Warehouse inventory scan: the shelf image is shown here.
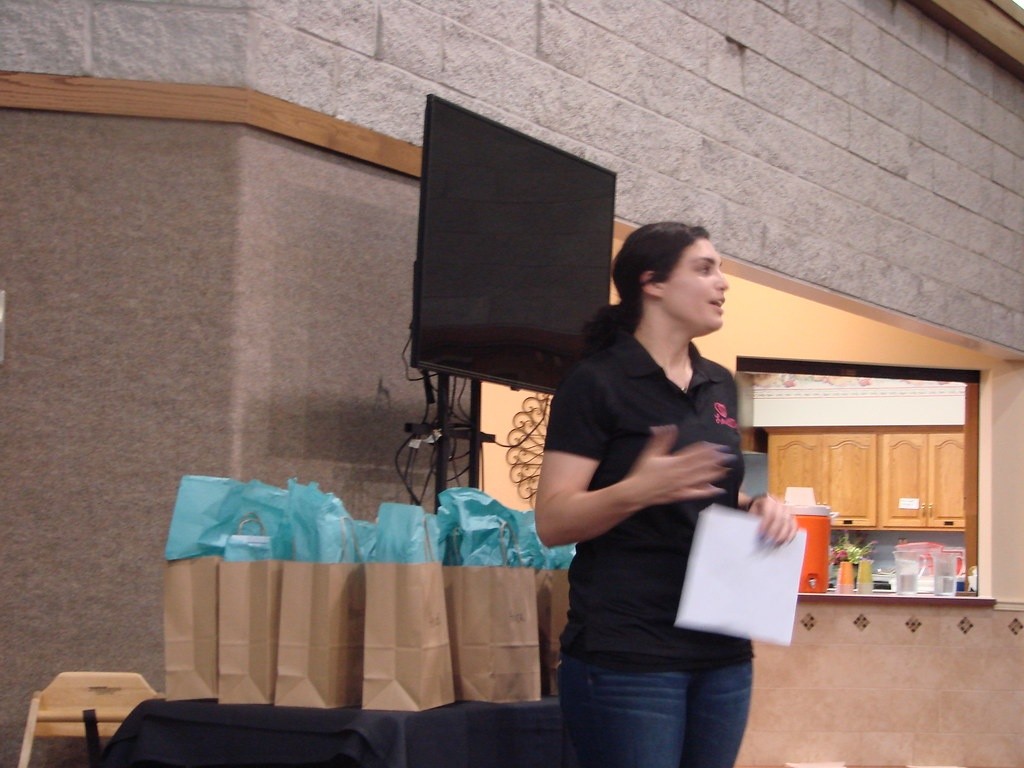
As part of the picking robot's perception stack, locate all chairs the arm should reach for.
[17,672,157,768]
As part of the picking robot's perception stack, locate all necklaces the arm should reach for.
[656,356,688,392]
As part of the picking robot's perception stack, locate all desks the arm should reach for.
[104,700,561,768]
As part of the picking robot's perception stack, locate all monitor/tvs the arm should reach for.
[409,93,617,395]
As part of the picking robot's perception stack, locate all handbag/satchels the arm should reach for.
[441,521,542,705]
[532,568,571,697]
[163,506,364,712]
[362,514,456,713]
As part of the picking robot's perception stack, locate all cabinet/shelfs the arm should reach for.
[767,424,968,533]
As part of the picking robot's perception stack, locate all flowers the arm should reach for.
[828,530,877,566]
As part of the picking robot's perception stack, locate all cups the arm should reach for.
[836,561,874,594]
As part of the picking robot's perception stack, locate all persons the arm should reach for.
[535,223,797,768]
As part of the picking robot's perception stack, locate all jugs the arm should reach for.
[931,553,964,595]
[894,542,943,594]
[892,551,928,596]
